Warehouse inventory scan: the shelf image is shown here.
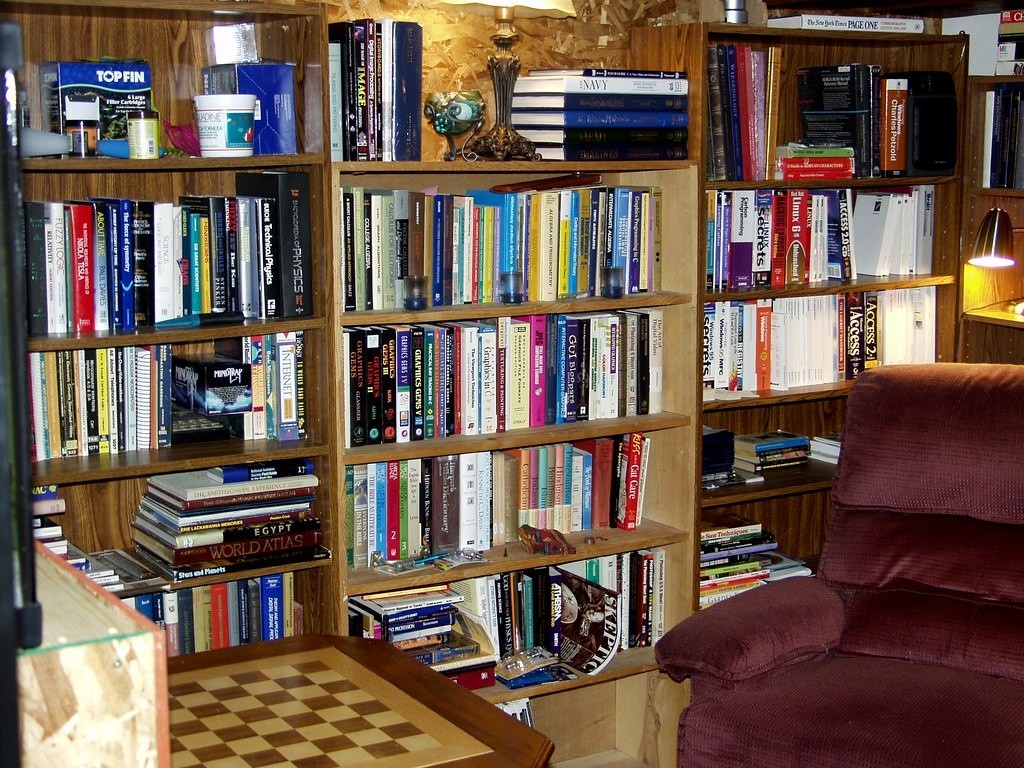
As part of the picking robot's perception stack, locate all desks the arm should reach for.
[166,634,555,768]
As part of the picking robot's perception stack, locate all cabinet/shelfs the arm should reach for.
[0,0,348,636]
[331,162,698,768]
[632,22,970,610]
[957,76,1024,364]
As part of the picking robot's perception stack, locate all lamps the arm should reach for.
[968,208,1024,316]
[428,0,577,161]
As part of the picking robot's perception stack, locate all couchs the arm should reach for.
[655,362,1024,768]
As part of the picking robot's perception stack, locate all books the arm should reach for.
[19,8,1024,691]
[528,68,687,80]
